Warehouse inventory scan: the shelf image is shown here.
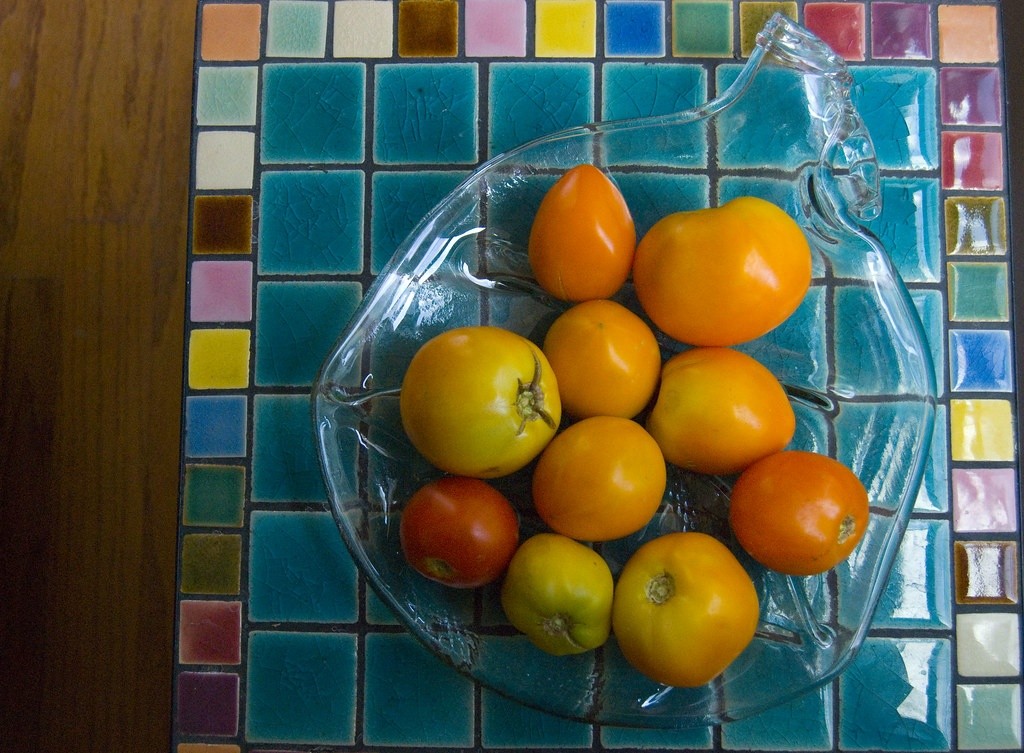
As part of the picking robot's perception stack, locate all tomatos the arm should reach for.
[401,159,869,691]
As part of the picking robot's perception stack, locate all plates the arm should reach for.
[311,14,943,727]
